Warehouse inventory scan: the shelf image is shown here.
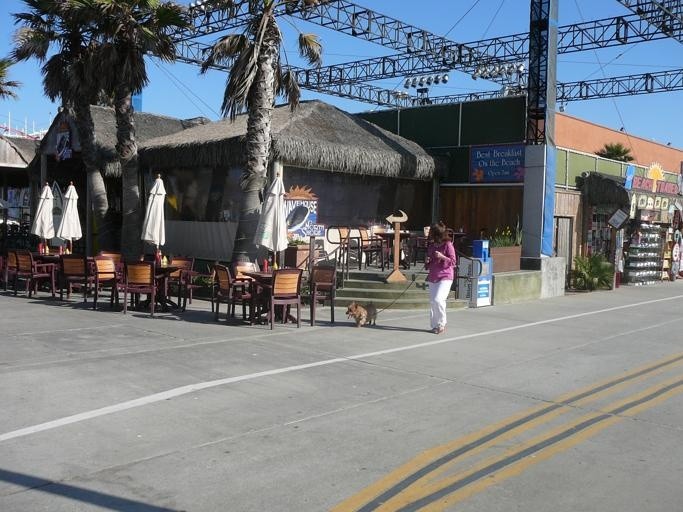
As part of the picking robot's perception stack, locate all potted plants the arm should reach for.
[489,214,523,273]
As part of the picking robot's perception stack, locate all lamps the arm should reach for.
[559,102,567,112]
[472,58,527,80]
[404,70,450,89]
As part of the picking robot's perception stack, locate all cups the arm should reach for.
[166,256,172,265]
[386,225,389,232]
[423,227,429,237]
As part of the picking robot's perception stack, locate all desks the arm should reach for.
[121,262,180,310]
[241,271,275,284]
[33,253,61,264]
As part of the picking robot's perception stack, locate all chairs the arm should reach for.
[13,249,56,298]
[233,262,261,281]
[99,250,123,268]
[124,261,163,318]
[166,257,195,307]
[301,266,338,326]
[250,267,303,330]
[4,249,30,291]
[215,264,253,325]
[93,255,134,310]
[326,225,485,271]
[59,254,96,304]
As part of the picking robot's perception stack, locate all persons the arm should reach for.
[424,220,456,334]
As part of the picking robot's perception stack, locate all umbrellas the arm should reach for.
[139,174,165,261]
[252,172,289,267]
[56,182,82,255]
[30,182,54,253]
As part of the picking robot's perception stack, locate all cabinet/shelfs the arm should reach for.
[622,221,666,285]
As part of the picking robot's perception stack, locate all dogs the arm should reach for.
[345,300,378,327]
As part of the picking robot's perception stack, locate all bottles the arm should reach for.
[153,250,160,265]
[38,242,69,255]
[162,255,167,265]
[272,262,276,270]
[262,258,267,272]
